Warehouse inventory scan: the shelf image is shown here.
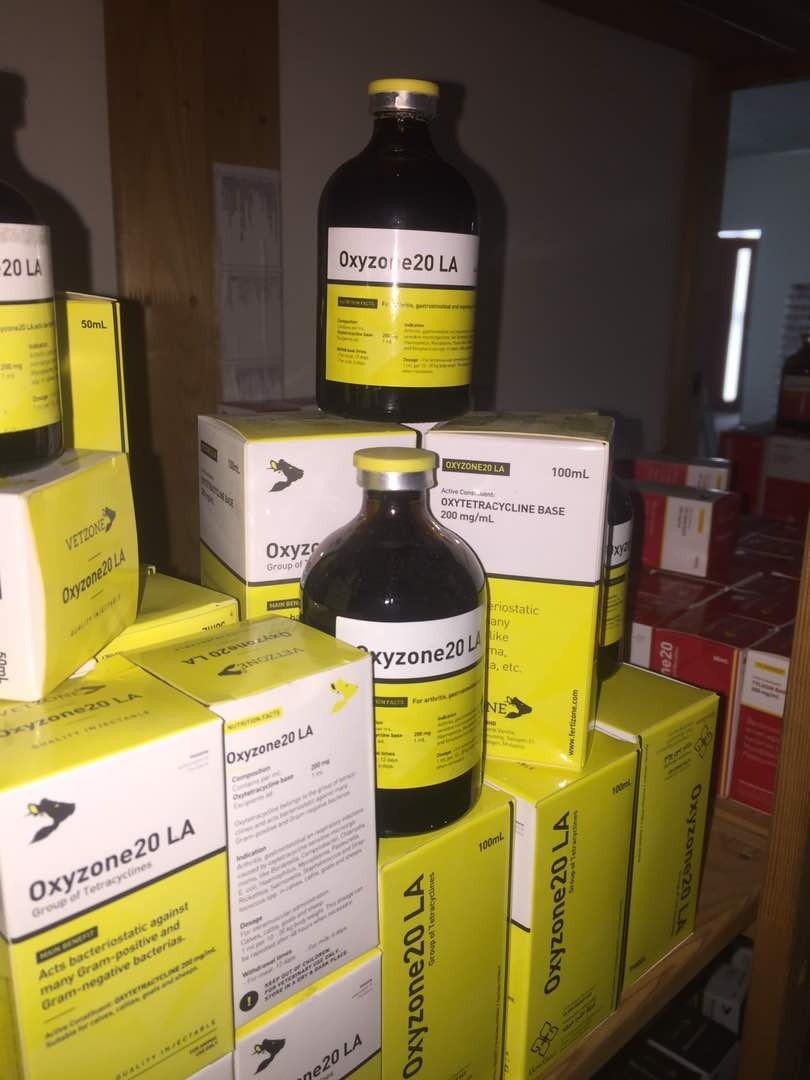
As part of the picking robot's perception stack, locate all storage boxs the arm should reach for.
[1,290,809,1079]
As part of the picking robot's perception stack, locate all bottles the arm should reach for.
[0,164,65,479]
[596,490,634,679]
[316,73,496,414]
[297,446,489,834]
[777,334,810,432]
